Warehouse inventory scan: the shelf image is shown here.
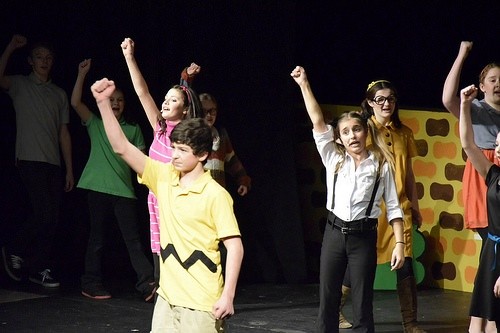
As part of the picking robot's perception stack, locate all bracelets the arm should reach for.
[396,242,404,244]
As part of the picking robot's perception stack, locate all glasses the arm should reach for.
[204,107,217,116]
[371,95,397,105]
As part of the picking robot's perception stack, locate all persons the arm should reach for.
[338,80,426,333]
[180,63,249,196]
[291,65,404,333]
[459,85,500,333]
[71,58,156,302]
[121,38,205,305]
[90,78,244,333]
[0,35,74,287]
[442,42,500,262]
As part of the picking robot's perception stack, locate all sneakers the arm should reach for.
[28,269,60,287]
[142,282,157,302]
[82,285,111,299]
[2,247,24,281]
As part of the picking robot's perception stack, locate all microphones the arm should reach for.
[161,110,163,112]
[347,144,350,146]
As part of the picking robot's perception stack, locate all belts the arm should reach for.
[328,218,377,235]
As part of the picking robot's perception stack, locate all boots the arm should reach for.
[338,285,353,329]
[397,276,427,333]
